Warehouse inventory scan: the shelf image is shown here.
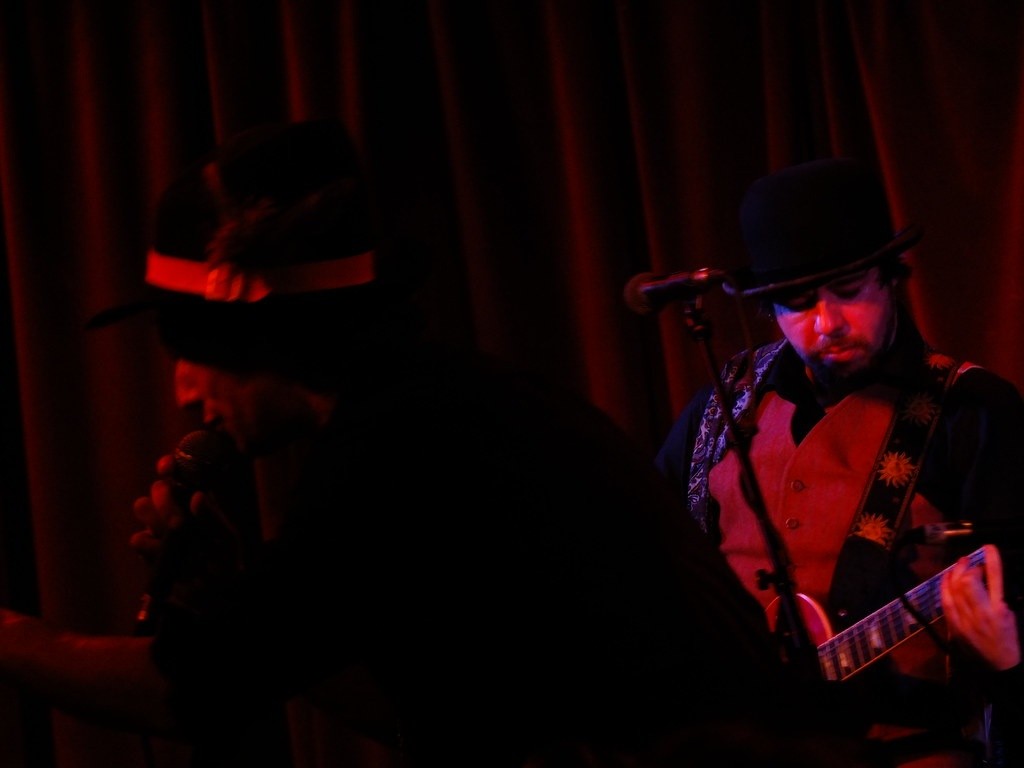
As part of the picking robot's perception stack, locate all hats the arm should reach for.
[146,121,424,390]
[720,157,924,301]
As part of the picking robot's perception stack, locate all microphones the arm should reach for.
[624,270,728,314]
[137,430,228,634]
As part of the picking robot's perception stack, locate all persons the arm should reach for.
[657,161,1024,766]
[1,119,775,766]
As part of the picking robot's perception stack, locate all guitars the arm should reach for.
[763,543,1004,684]
